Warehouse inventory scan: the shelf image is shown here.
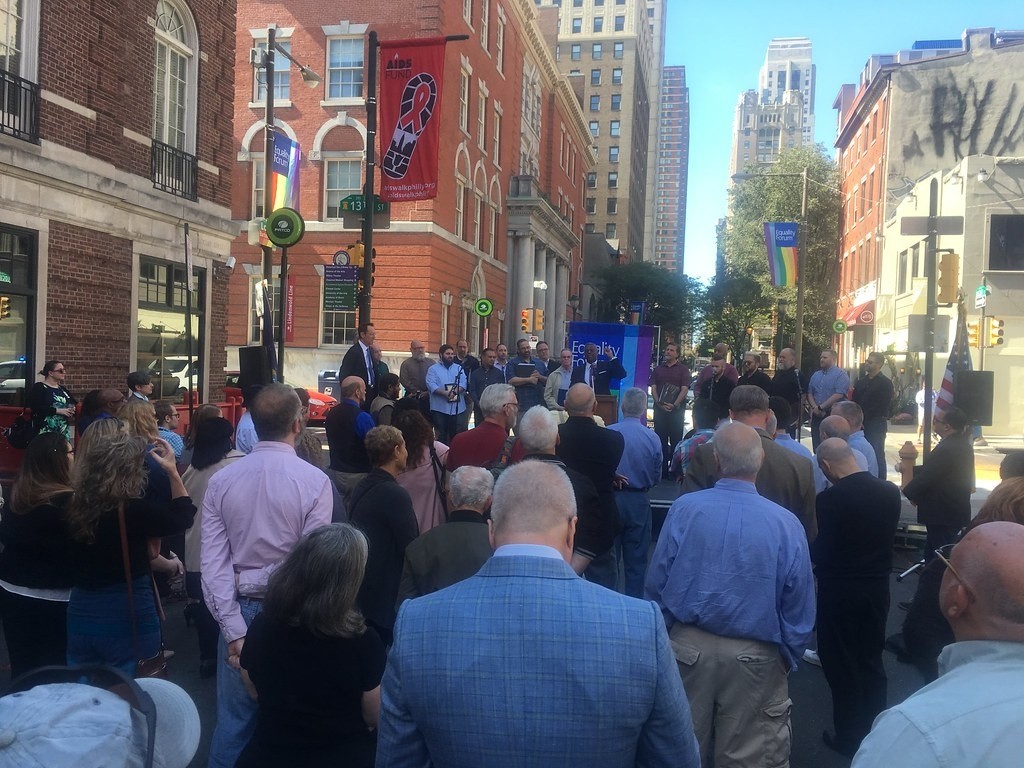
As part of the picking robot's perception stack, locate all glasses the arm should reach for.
[503,403,521,411]
[743,360,757,365]
[111,396,125,402]
[933,415,953,428]
[865,359,880,365]
[63,448,76,455]
[0,664,156,768]
[935,544,975,603]
[52,368,66,373]
[170,414,180,418]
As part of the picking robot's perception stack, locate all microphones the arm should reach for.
[460,358,468,365]
[794,369,799,374]
[591,362,596,376]
[712,367,717,375]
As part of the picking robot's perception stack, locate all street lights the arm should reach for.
[730,170,810,371]
[746,326,753,351]
[261,43,324,345]
[777,298,789,356]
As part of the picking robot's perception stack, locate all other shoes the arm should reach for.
[898,602,912,612]
[973,439,988,446]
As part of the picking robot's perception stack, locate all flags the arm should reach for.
[933,303,973,430]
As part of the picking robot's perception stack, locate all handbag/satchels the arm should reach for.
[437,462,448,518]
[490,436,517,486]
[6,383,52,448]
[138,652,168,681]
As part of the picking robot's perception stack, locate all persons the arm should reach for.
[0,322,1024,768]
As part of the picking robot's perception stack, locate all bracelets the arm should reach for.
[673,403,678,408]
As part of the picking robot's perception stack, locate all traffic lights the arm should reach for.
[991,318,1005,346]
[521,310,530,333]
[354,243,376,287]
[968,319,980,347]
[938,253,960,302]
[535,309,546,330]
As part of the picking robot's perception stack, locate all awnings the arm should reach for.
[841,299,874,331]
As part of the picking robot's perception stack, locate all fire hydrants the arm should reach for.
[894,440,919,491]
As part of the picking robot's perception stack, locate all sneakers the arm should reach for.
[802,648,823,667]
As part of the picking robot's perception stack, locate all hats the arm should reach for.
[0,677,201,768]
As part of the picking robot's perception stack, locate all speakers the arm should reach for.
[954,370,993,426]
[239,346,272,389]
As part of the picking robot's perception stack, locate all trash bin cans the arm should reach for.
[317,369,341,403]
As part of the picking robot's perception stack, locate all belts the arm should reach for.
[614,486,649,493]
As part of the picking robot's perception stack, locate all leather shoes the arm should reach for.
[823,729,854,757]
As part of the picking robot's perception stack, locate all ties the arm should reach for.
[589,365,594,388]
[367,348,375,385]
[502,365,505,381]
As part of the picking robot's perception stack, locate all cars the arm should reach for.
[686,380,697,401]
[184,371,335,427]
[0,361,26,394]
[147,355,198,403]
